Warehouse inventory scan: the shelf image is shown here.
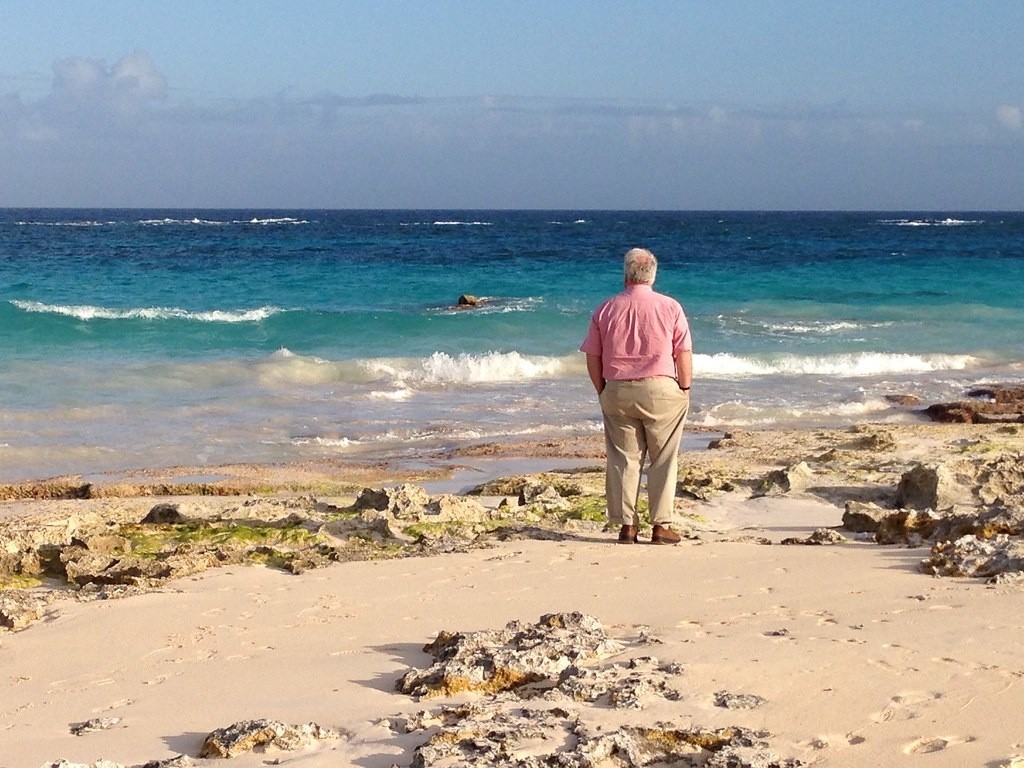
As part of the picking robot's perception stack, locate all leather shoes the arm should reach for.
[652,525,681,544]
[619,525,639,543]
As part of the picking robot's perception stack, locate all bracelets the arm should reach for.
[680,386,691,390]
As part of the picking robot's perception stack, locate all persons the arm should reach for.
[580,246,693,544]
